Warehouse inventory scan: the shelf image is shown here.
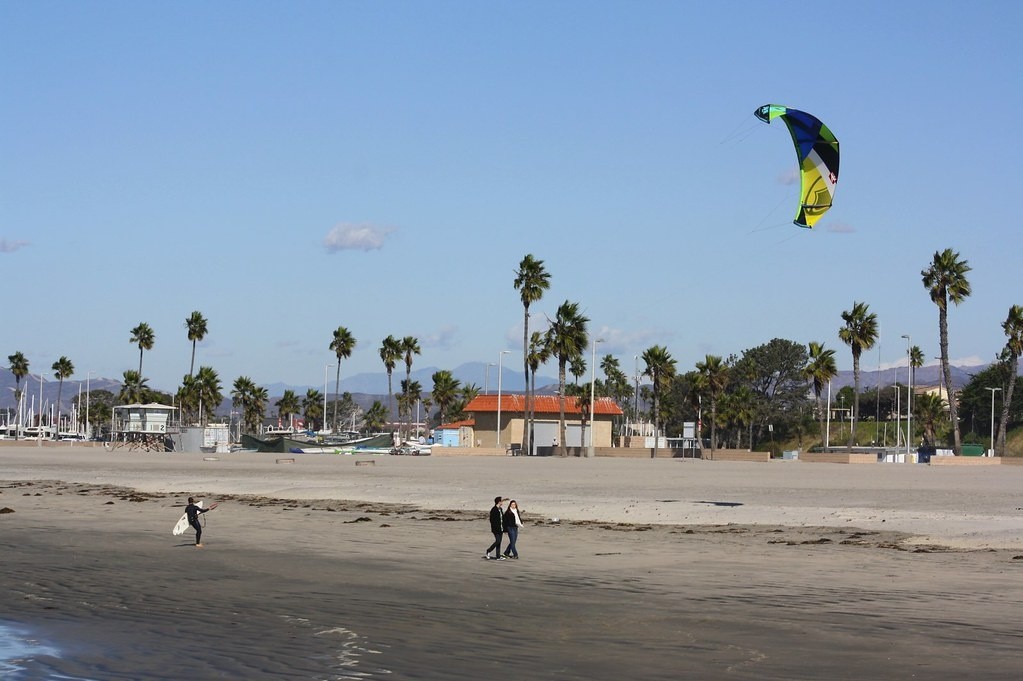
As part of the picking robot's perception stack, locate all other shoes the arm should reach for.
[502,554,510,558]
[511,556,518,559]
[485,552,491,560]
[196,544,202,547]
[496,556,506,561]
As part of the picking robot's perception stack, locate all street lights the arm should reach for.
[901,335,911,454]
[590,339,608,447]
[485,363,497,395]
[891,386,900,448]
[86,372,95,436]
[497,350,511,444]
[38,373,48,445]
[324,365,335,430]
[985,387,1002,457]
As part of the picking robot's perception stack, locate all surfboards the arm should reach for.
[172,501,205,537]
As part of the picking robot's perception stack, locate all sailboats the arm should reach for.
[0,380,87,442]
[343,412,360,439]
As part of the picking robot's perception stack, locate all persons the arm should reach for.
[418,435,434,445]
[484,497,507,560]
[185,497,210,547]
[502,500,524,559]
[552,437,559,447]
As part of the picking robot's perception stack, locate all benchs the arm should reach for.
[506,444,522,456]
